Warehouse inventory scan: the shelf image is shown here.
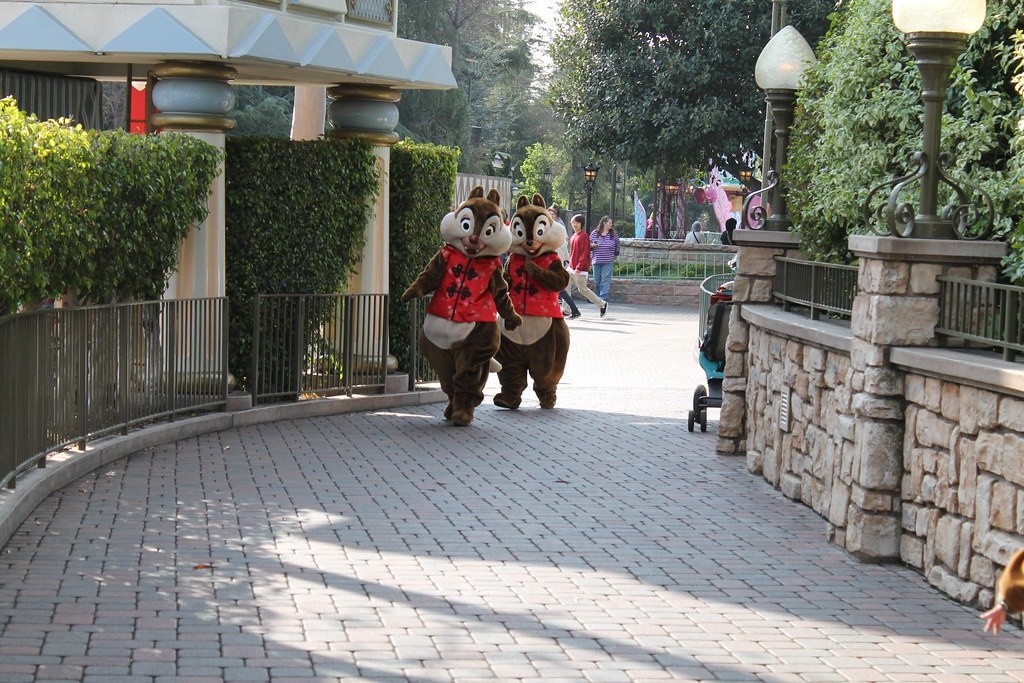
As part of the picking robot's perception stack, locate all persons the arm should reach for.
[587,216,620,304]
[499,207,581,319]
[648,215,658,229]
[562,215,608,318]
[685,221,706,243]
[720,218,737,245]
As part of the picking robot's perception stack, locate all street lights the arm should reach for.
[664,179,680,240]
[737,163,754,229]
[583,162,599,236]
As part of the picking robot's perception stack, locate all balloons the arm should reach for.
[692,187,717,204]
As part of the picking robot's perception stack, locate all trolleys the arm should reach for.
[688,273,738,432]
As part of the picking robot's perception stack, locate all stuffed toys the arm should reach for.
[402,186,569,425]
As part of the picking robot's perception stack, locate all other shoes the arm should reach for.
[599,302,608,318]
[565,313,581,320]
[562,311,570,317]
